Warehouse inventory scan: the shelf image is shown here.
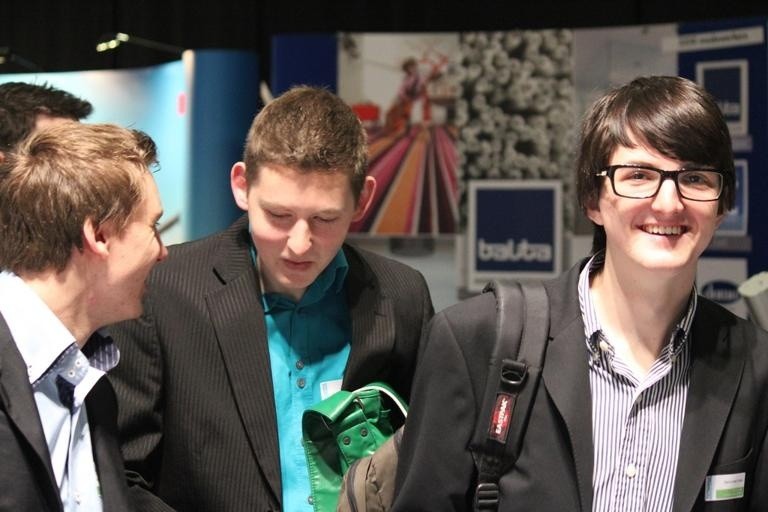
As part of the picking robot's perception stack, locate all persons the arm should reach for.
[0,123,169,512]
[100,84,434,512]
[395,73,768,510]
[0,81,93,164]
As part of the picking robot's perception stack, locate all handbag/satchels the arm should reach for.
[300,380,410,512]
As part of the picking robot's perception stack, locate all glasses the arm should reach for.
[596,163,724,203]
